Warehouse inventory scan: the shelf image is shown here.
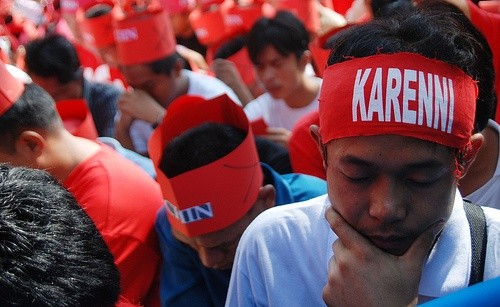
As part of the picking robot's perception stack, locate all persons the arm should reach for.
[146,93,328,307]
[1,163,122,307]
[224,13,500,307]
[0,0,500,210]
[1,63,163,307]
